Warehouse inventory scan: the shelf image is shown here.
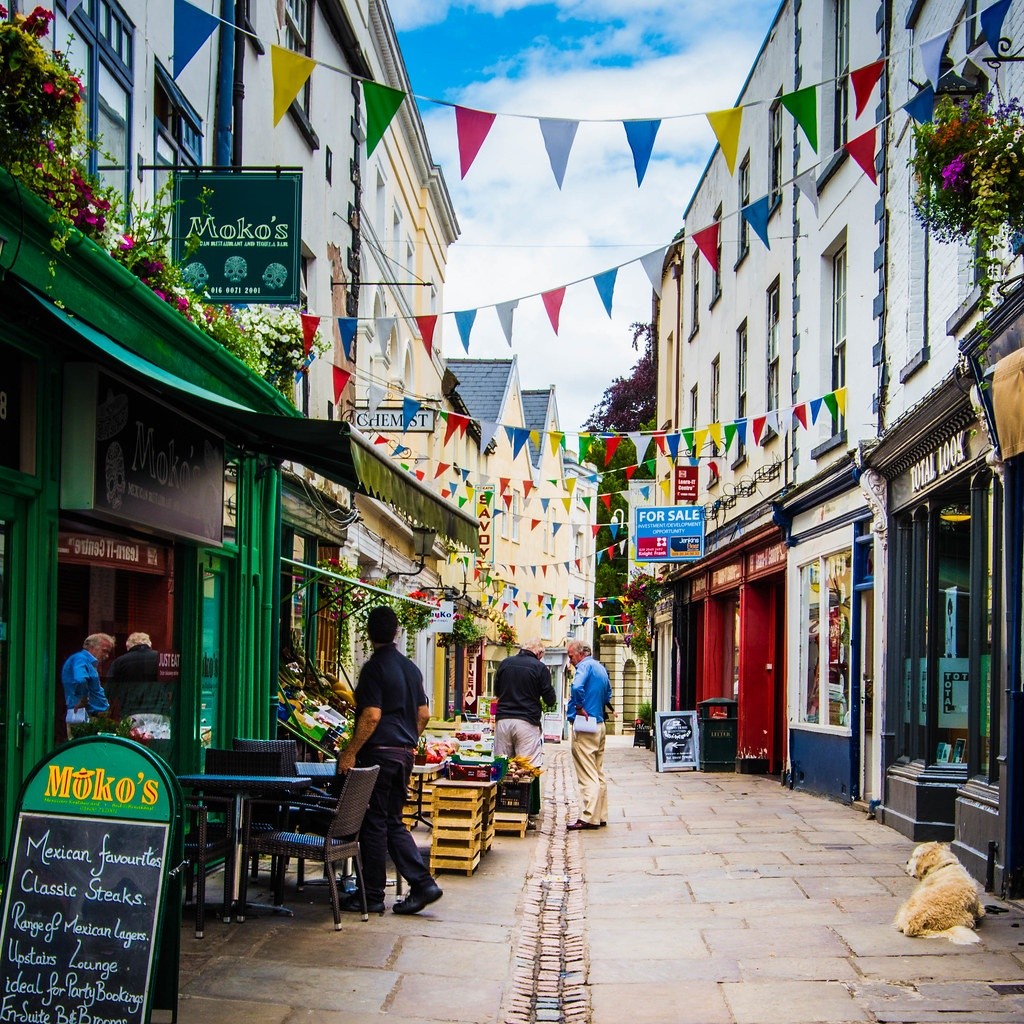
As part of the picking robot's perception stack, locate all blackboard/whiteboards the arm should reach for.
[0,811,179,1023]
[634,723,650,746]
[655,711,700,767]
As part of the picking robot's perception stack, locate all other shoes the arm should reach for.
[528,813,540,821]
[331,893,386,913]
[392,886,444,915]
[566,819,599,830]
[600,821,607,827]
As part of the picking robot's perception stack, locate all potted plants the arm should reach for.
[415,736,428,766]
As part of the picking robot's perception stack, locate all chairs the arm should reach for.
[181,736,380,931]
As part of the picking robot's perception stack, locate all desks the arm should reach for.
[403,760,445,831]
[180,774,311,921]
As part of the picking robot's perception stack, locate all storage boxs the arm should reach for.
[495,781,534,813]
[278,688,355,756]
[446,762,492,782]
[451,753,510,778]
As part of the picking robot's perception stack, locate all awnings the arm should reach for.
[16,279,482,556]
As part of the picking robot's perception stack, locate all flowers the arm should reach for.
[904,90,1024,455]
[0,4,333,405]
[622,566,663,681]
[295,557,485,679]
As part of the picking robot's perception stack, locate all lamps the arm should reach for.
[940,505,971,522]
[387,523,438,580]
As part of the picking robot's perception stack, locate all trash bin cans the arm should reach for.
[697,697,738,773]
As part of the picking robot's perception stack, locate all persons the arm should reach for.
[492,638,556,829]
[59,632,115,740]
[563,642,612,830]
[604,703,619,721]
[337,605,443,916]
[106,631,178,713]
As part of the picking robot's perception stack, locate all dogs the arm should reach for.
[894,841,986,946]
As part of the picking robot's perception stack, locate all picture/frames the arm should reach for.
[951,739,967,763]
